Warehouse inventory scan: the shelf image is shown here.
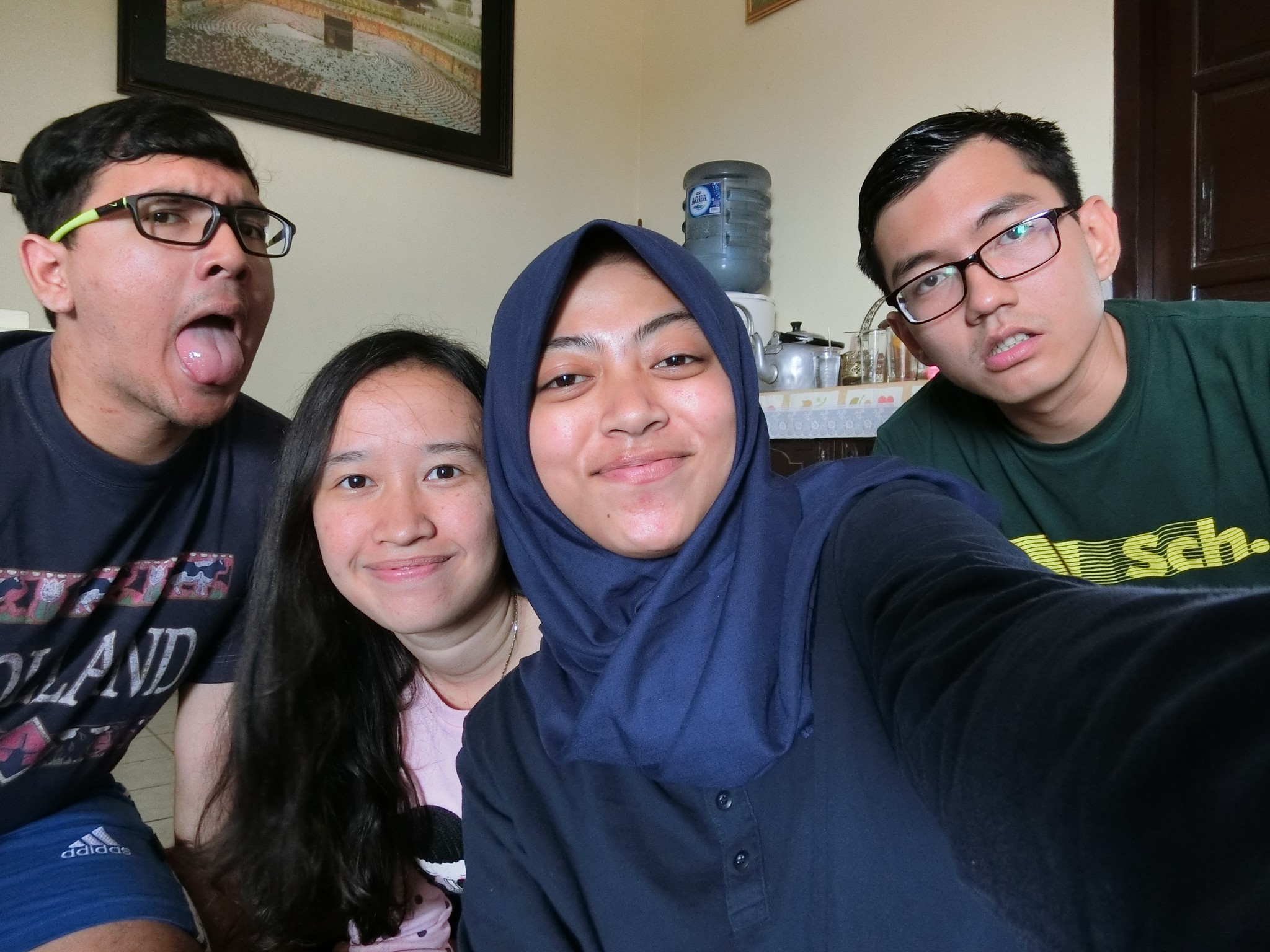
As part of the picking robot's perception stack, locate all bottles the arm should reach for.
[679,161,770,291]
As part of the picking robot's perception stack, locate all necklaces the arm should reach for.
[493,585,525,683]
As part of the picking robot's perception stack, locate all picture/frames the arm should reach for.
[113,0,515,180]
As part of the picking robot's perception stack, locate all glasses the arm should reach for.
[48,192,297,262]
[884,204,1079,326]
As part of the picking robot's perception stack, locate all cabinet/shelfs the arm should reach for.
[755,378,939,481]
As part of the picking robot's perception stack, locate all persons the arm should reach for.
[855,105,1268,592]
[454,221,1268,952]
[2,96,306,950]
[198,322,554,950]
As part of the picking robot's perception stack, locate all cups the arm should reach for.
[861,330,892,384]
[811,346,844,389]
[888,328,920,381]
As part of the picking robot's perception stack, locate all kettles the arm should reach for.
[750,322,845,391]
